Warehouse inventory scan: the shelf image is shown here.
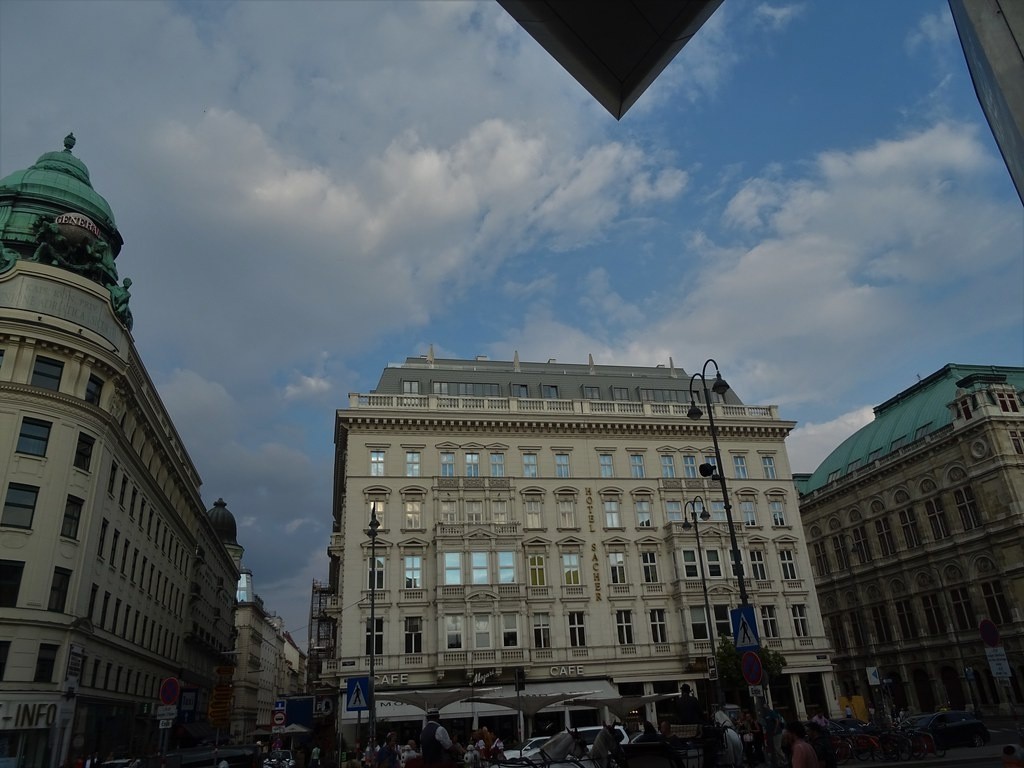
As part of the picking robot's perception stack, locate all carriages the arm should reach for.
[404,720,626,768]
[620,706,744,768]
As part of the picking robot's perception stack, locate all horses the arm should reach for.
[711,704,743,768]
[540,718,625,768]
[487,726,589,768]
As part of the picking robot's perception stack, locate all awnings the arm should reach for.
[185,721,218,737]
[340,679,622,724]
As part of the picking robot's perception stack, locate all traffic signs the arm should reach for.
[208,666,234,725]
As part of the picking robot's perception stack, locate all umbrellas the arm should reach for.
[247,728,272,735]
[285,723,312,750]
[371,689,680,736]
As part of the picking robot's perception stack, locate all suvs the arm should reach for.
[262,750,296,768]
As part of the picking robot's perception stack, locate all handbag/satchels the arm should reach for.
[318,759,320,765]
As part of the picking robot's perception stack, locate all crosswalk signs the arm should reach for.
[346,677,370,712]
[729,606,761,651]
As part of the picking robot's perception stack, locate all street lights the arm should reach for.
[682,495,725,710]
[367,501,380,751]
[686,359,749,604]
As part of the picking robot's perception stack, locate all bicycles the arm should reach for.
[831,722,945,764]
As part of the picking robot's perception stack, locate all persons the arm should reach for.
[807,722,837,768]
[311,744,321,768]
[845,705,852,718]
[674,684,704,722]
[660,721,681,749]
[452,734,461,751]
[472,729,507,768]
[783,721,819,768]
[107,752,115,761]
[812,711,829,727]
[63,752,102,768]
[376,732,402,768]
[731,706,787,768]
[891,705,911,723]
[422,708,456,764]
[633,721,662,743]
[401,740,422,762]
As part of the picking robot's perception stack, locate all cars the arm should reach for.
[900,711,991,750]
[801,717,881,735]
[503,726,694,760]
[99,745,262,768]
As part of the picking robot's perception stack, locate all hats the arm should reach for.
[424,707,441,716]
